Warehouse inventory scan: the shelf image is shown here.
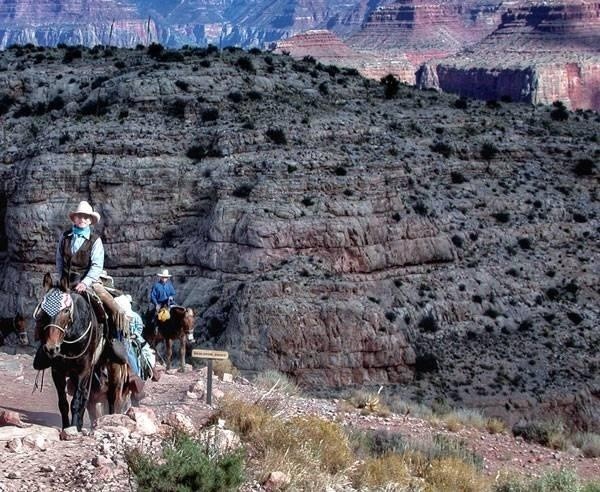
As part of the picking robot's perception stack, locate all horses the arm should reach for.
[142,304,196,374]
[40,269,124,432]
[0,312,29,346]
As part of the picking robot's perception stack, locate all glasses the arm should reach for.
[77,215,88,219]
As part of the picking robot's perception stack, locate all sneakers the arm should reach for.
[112,338,129,364]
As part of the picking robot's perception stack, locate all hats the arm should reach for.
[114,294,133,311]
[69,201,101,223]
[157,269,172,278]
[100,270,113,286]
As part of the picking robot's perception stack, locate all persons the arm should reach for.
[149,269,178,336]
[54,200,160,399]
